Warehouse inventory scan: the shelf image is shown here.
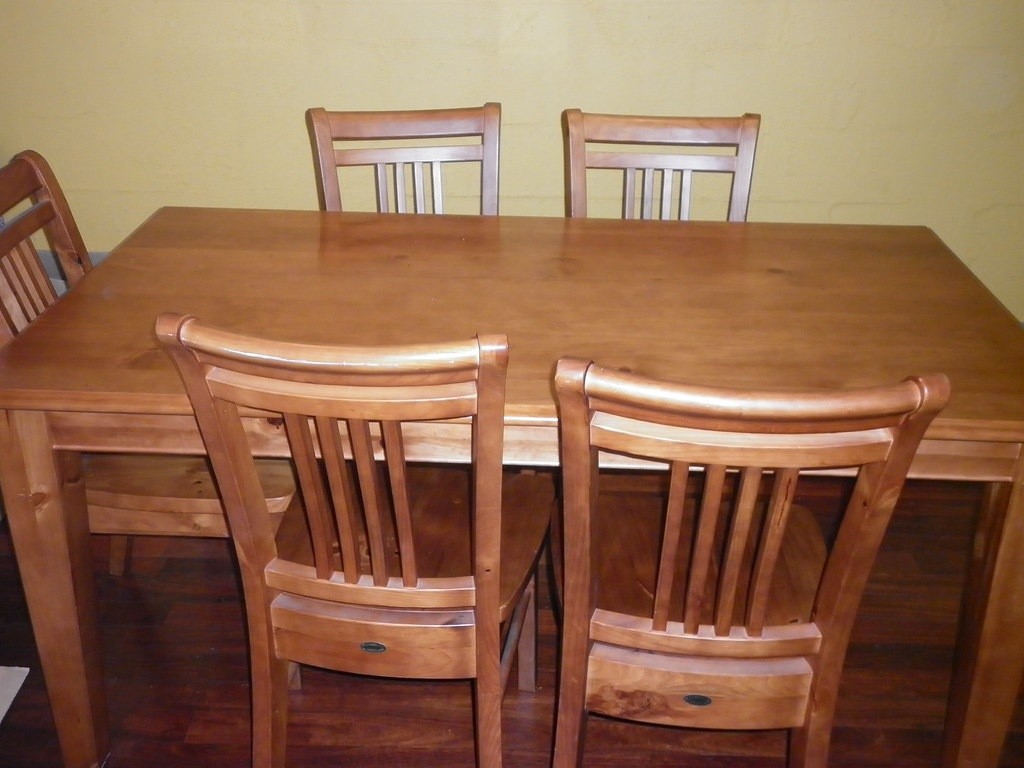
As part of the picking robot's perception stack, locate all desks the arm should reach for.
[0,204,1024,768]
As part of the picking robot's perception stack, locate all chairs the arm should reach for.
[146,310,558,768]
[555,109,762,223]
[544,352,957,768]
[303,100,509,214]
[0,147,303,698]
